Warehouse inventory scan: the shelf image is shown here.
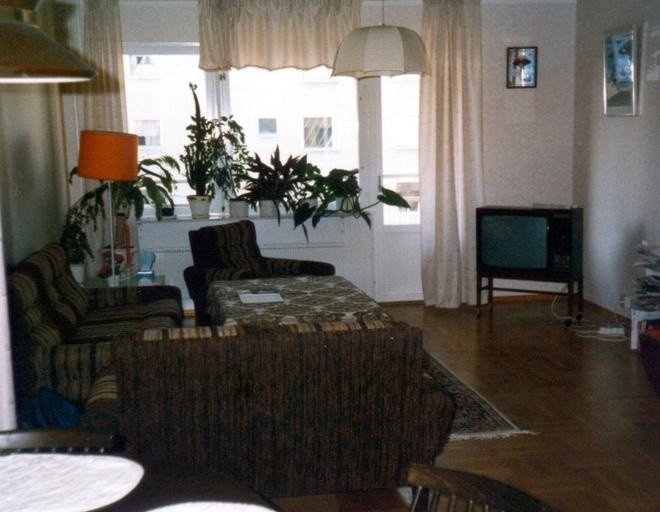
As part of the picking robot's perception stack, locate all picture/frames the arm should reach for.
[600,24,640,118]
[505,46,538,90]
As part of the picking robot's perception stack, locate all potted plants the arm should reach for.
[61,154,181,283]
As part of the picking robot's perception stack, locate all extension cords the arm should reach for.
[599,325,624,334]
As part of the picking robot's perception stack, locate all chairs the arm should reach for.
[183,219,336,326]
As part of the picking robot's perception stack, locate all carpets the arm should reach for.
[423,348,543,442]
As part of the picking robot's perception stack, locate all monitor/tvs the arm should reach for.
[476,206,583,280]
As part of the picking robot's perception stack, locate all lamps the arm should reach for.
[77,129,140,281]
[329,0,434,82]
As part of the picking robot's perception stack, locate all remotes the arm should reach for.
[533,203,572,210]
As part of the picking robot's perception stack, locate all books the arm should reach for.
[632,239,659,314]
